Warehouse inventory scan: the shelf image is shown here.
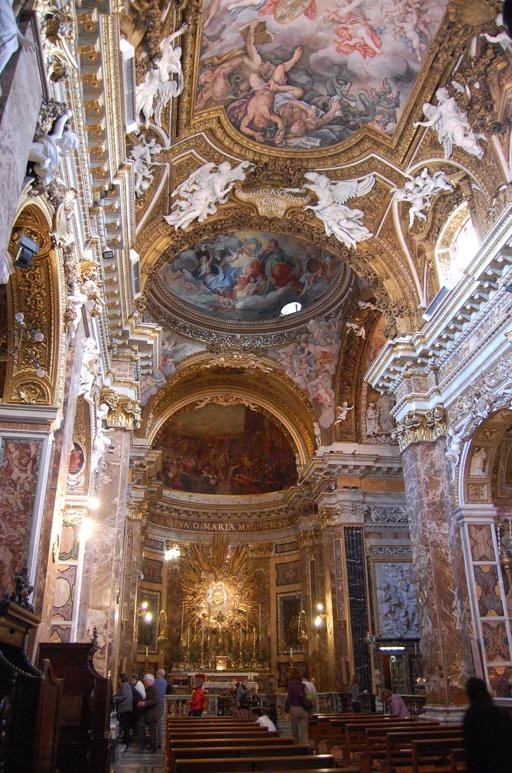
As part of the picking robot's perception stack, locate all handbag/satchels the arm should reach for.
[203,698,210,710]
[303,687,317,708]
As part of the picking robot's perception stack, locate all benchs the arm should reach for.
[163,710,469,771]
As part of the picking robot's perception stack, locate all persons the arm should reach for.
[1,493,41,597]
[412,86,487,161]
[374,560,421,639]
[459,674,511,770]
[111,667,410,753]
